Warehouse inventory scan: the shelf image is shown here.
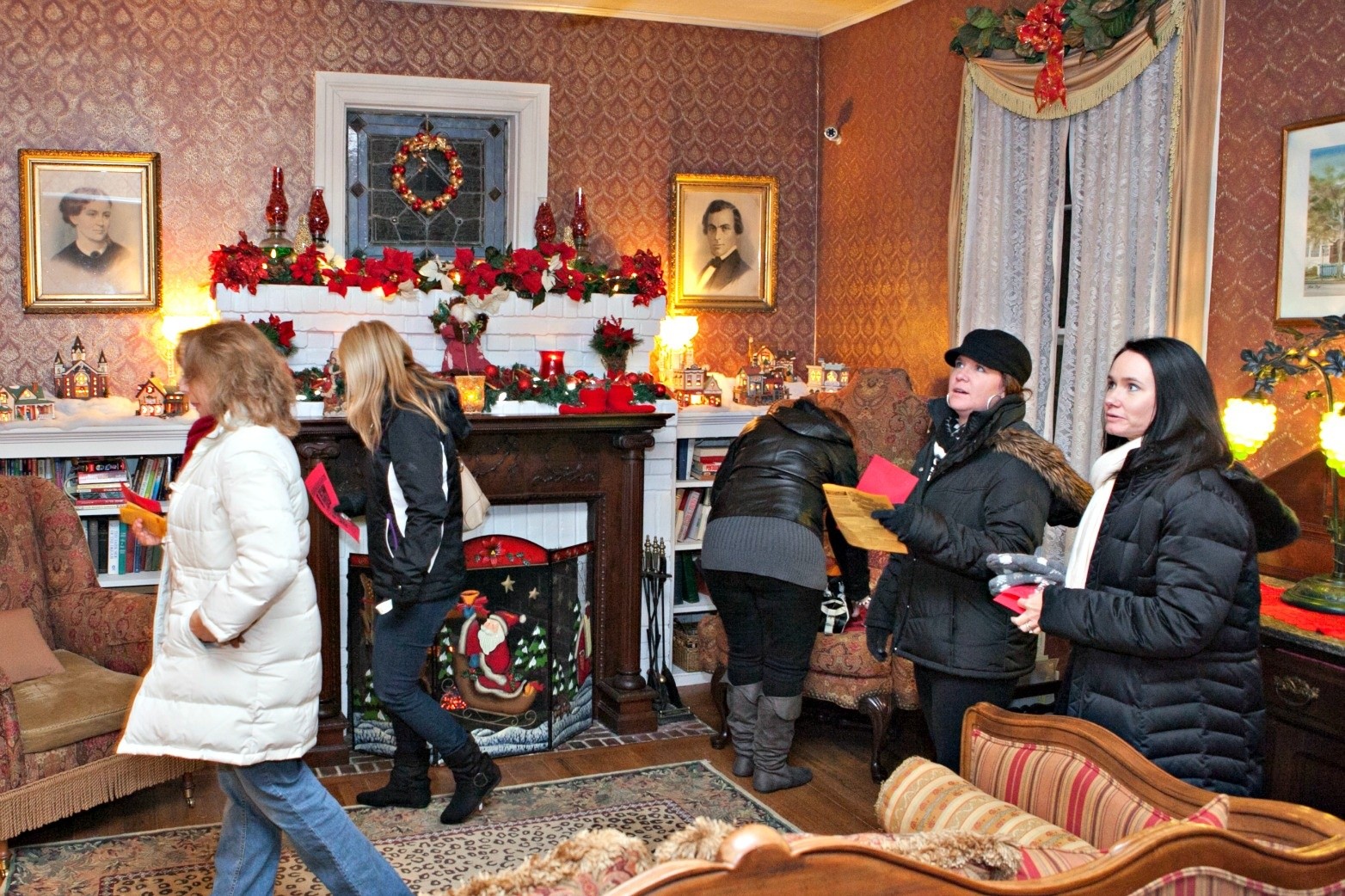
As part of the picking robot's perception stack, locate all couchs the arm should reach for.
[605,700,1345,896]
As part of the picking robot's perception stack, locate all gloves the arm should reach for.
[866,628,890,662]
[986,546,1068,579]
[872,503,914,538]
[989,572,1057,595]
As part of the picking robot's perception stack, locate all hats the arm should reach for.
[945,328,1033,388]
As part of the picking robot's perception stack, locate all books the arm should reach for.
[82,520,164,575]
[674,552,708,605]
[0,456,180,510]
[676,488,712,544]
[676,438,735,481]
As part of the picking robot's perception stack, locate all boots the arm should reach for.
[753,688,813,792]
[719,669,763,777]
[441,730,501,824]
[356,748,432,808]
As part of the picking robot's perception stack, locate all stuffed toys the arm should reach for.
[440,298,488,373]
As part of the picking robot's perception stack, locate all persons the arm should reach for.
[51,187,130,274]
[332,320,503,825]
[698,199,751,290]
[701,397,870,793]
[117,318,412,896]
[1011,337,1301,797]
[864,329,1093,770]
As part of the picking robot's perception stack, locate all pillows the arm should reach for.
[432,757,1234,896]
[0,607,66,683]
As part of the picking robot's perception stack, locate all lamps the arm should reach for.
[1219,315,1345,616]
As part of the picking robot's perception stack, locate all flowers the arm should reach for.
[204,228,670,310]
[241,312,299,360]
[590,316,644,359]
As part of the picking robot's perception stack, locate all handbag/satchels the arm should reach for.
[457,455,490,533]
[817,576,853,636]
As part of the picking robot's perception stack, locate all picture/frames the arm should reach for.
[17,147,163,315]
[1274,113,1345,327]
[665,173,781,314]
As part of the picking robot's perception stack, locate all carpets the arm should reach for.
[0,759,805,896]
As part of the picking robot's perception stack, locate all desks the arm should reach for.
[1009,656,1063,717]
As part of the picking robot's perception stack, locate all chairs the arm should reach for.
[0,474,210,878]
[696,367,921,785]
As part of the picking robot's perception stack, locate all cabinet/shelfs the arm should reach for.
[673,414,766,686]
[0,440,190,597]
[1257,643,1345,821]
